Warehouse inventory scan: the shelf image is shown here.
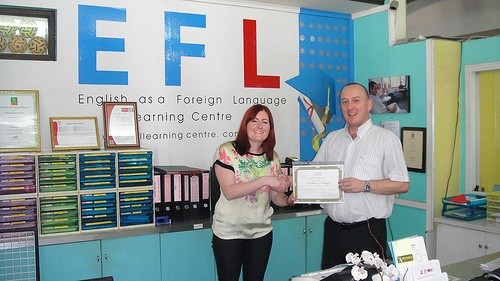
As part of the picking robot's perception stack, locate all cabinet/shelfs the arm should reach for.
[38,209,329,281]
[433,216,500,266]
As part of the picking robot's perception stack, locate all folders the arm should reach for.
[154,165,201,175]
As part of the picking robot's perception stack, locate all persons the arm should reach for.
[312,83,410,272]
[369,81,406,113]
[210,104,296,281]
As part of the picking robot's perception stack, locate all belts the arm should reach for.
[330,218,377,233]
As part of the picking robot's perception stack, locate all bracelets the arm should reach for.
[286,196,294,206]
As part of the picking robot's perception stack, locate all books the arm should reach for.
[388,236,448,281]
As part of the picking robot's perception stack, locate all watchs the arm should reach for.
[364,180,371,193]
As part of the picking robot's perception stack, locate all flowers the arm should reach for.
[346,250,400,281]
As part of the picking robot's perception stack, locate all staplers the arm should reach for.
[156,216,171,225]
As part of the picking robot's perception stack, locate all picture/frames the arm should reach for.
[0,4,58,62]
